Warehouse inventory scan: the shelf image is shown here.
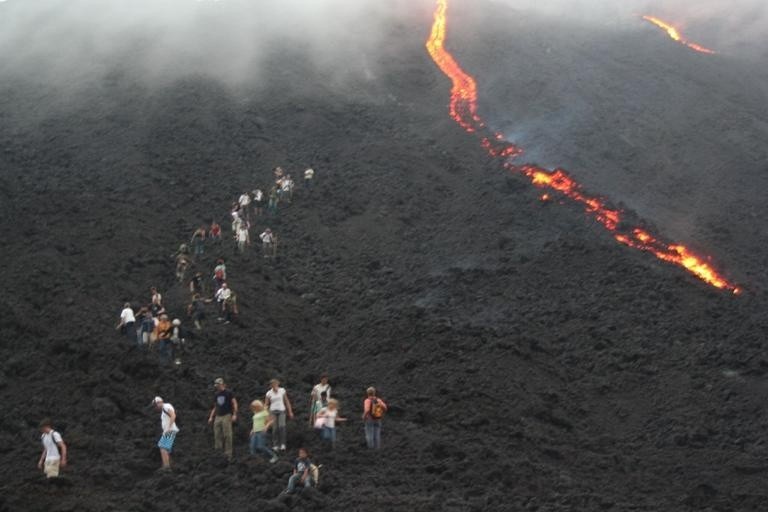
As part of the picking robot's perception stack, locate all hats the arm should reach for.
[214,378,224,387]
[39,417,50,427]
[152,396,162,404]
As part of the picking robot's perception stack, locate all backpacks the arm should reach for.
[216,268,222,280]
[369,396,384,419]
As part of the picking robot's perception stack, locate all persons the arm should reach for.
[36,421,68,479]
[209,375,391,495]
[117,165,316,365]
[152,395,180,475]
